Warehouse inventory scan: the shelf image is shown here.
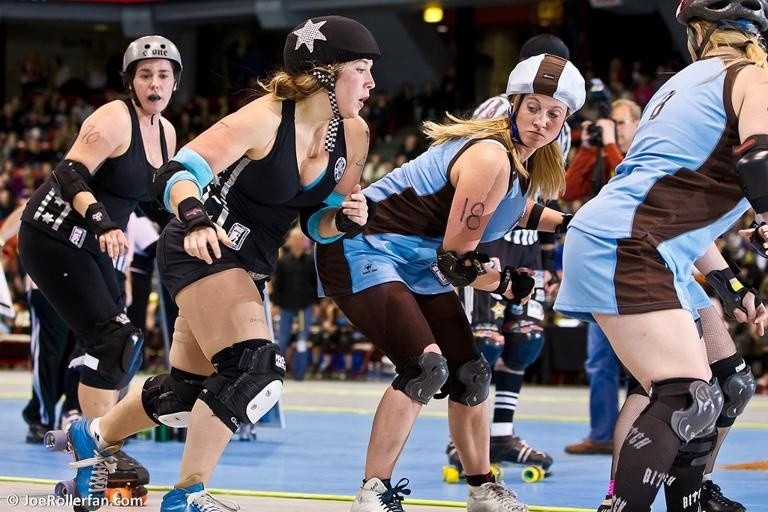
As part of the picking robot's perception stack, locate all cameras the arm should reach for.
[586,78,613,147]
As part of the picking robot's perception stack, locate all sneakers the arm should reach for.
[701,481,746,512]
[565,439,613,454]
[467,482,529,512]
[161,474,240,512]
[350,477,410,512]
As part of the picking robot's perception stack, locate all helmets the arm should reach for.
[122,36,183,89]
[284,16,381,76]
[676,0,768,31]
[506,35,586,113]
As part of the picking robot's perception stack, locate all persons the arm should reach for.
[0,0,768,512]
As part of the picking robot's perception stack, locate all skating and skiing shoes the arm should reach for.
[27,409,149,512]
[442,435,553,483]
